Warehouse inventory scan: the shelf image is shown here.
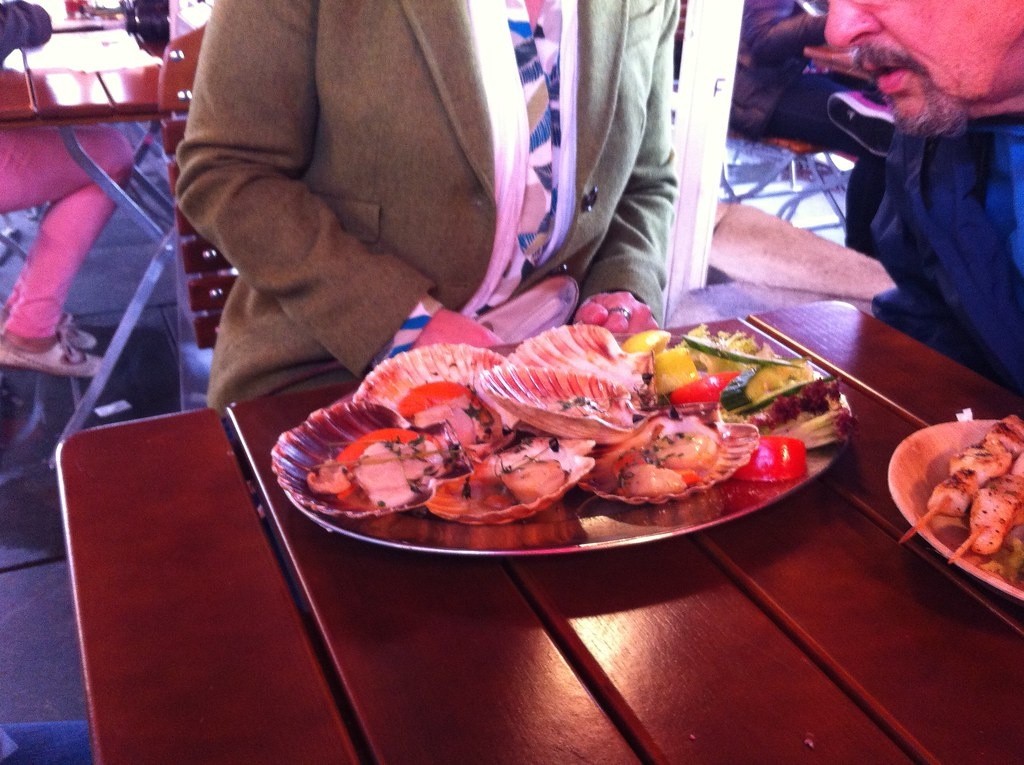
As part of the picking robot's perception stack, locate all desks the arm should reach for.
[53,298,1024,764]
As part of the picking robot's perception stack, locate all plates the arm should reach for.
[270,363,851,556]
[888,418,1024,601]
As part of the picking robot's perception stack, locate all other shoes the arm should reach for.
[0,331,101,377]
[56,311,97,350]
[827,90,898,155]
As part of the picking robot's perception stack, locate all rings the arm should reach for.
[608,306,633,320]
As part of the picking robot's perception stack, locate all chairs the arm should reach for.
[156,18,239,347]
[0,29,169,471]
[715,131,848,244]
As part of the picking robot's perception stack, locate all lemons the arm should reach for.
[655,348,699,395]
[621,330,672,356]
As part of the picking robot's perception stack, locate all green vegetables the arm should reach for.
[750,377,864,447]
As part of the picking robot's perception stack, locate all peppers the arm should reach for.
[729,436,810,484]
[673,373,738,408]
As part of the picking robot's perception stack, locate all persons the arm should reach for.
[174,0,680,420]
[730,0,1024,399]
[0,0,133,378]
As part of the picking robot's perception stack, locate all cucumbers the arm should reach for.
[683,333,841,416]
